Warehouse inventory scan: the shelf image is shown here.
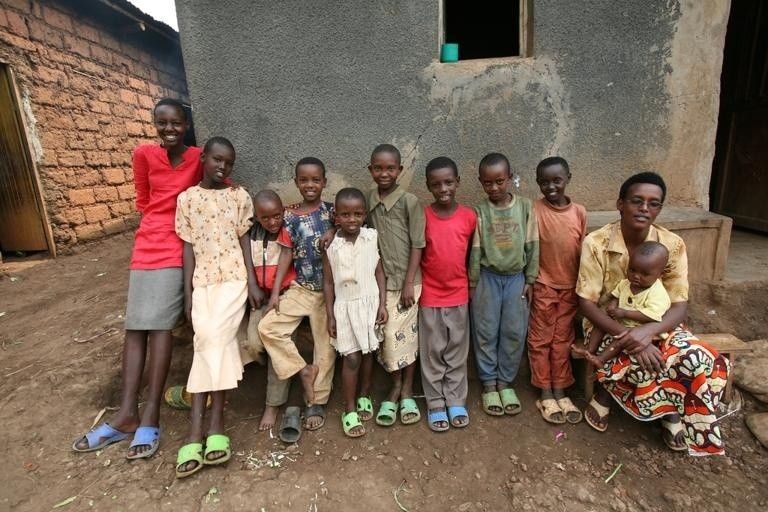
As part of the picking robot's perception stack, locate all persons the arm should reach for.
[255,155,337,432]
[468,152,541,417]
[570,170,732,456]
[530,154,587,426]
[417,156,479,432]
[317,143,428,427]
[569,240,674,370]
[173,136,267,479]
[71,98,233,461]
[320,187,391,438]
[243,187,296,432]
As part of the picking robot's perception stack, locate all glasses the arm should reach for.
[625,197,663,210]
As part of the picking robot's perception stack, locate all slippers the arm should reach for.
[535,399,566,424]
[305,404,326,430]
[499,389,521,414]
[279,407,301,443]
[165,386,212,409]
[357,395,373,421]
[342,412,365,437]
[73,422,134,452]
[557,397,582,424]
[204,435,230,464]
[176,443,203,479]
[661,417,687,450]
[400,399,420,424]
[481,392,504,416]
[376,401,399,426]
[585,395,610,432]
[428,407,449,431]
[127,427,160,459]
[448,406,468,427]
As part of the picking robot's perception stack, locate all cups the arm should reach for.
[440,42,459,63]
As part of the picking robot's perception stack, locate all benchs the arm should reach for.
[571,332,754,404]
